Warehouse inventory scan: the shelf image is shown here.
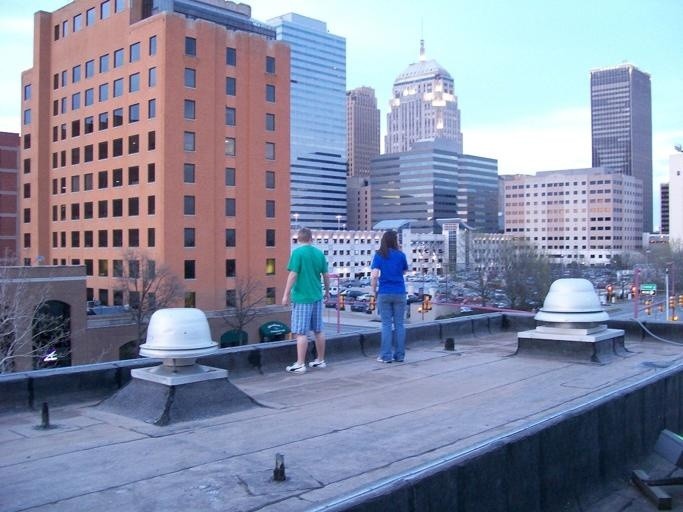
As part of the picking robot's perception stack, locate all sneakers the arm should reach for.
[375,354,404,363]
[307,358,326,368]
[284,361,306,373]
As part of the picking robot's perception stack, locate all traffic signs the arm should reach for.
[641,290,656,296]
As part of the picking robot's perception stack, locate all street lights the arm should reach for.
[293,214,299,229]
[336,215,345,230]
[665,267,669,320]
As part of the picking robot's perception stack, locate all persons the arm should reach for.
[369,230,408,364]
[280,226,331,373]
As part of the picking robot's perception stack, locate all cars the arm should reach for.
[403,270,634,312]
[322,276,378,312]
